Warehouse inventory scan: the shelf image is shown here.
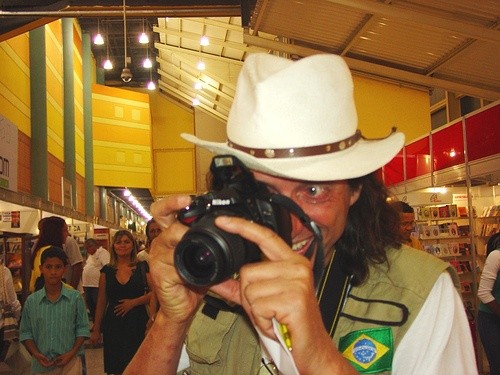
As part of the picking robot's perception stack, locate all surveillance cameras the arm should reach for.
[121,69,132,82]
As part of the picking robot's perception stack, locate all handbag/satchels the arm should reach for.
[4,308,19,342]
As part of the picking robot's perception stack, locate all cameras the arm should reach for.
[174,154,293,288]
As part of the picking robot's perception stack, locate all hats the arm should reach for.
[180,51,406,182]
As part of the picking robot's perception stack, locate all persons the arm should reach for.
[1,215,165,375]
[392,200,426,252]
[477,248,500,375]
[119,52,480,375]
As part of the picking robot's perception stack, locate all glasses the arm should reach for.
[256,357,278,374]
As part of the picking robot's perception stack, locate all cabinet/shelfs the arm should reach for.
[412,204,478,322]
[473,215,500,270]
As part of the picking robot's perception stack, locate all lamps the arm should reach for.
[143,43,152,69]
[94,17,104,45]
[104,22,113,69]
[139,18,149,43]
[147,68,155,91]
